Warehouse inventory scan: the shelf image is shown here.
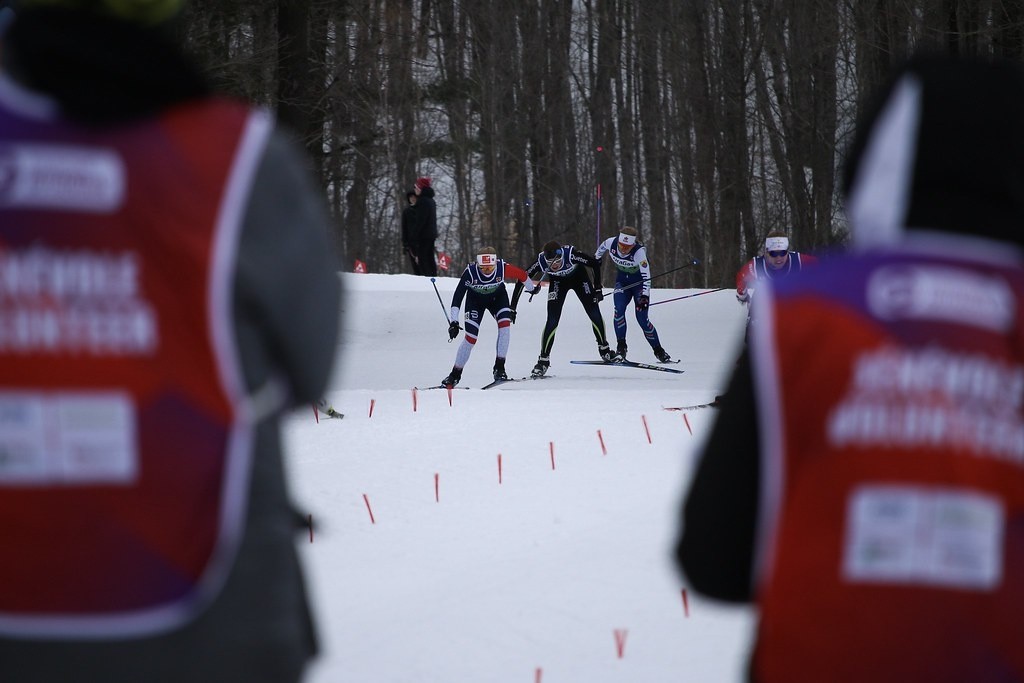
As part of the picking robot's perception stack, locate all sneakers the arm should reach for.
[441,365,463,388]
[531,353,550,378]
[654,348,671,364]
[615,343,628,358]
[492,358,507,381]
[597,342,616,363]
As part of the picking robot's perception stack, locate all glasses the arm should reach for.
[479,266,496,270]
[767,250,789,257]
[618,242,635,249]
[544,248,564,265]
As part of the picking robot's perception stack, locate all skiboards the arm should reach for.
[414,378,524,392]
[517,360,685,384]
[662,403,713,412]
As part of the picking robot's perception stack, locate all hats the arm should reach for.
[415,176,431,191]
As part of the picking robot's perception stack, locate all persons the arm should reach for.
[441,246,541,387]
[594,226,671,362]
[0,0,345,683]
[401,178,437,277]
[736,231,819,307]
[677,50,1024,683]
[510,241,615,377]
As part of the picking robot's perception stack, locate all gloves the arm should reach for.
[509,307,518,324]
[593,289,604,303]
[529,285,541,295]
[736,292,750,305]
[448,320,464,339]
[636,295,650,312]
